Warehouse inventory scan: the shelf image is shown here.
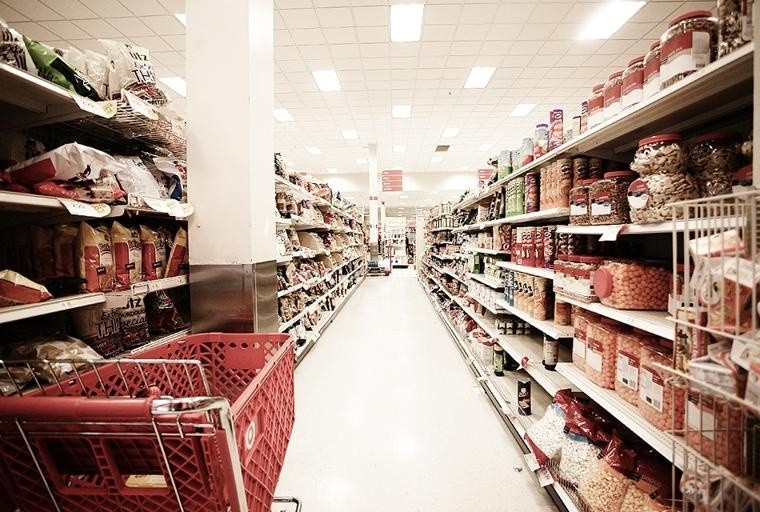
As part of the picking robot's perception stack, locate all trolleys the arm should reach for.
[366,244,392,276]
[0,329,301,512]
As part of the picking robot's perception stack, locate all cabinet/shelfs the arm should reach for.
[435,147,557,422]
[557,45,760,511]
[274,174,371,370]
[418,210,452,283]
[5,57,189,401]
